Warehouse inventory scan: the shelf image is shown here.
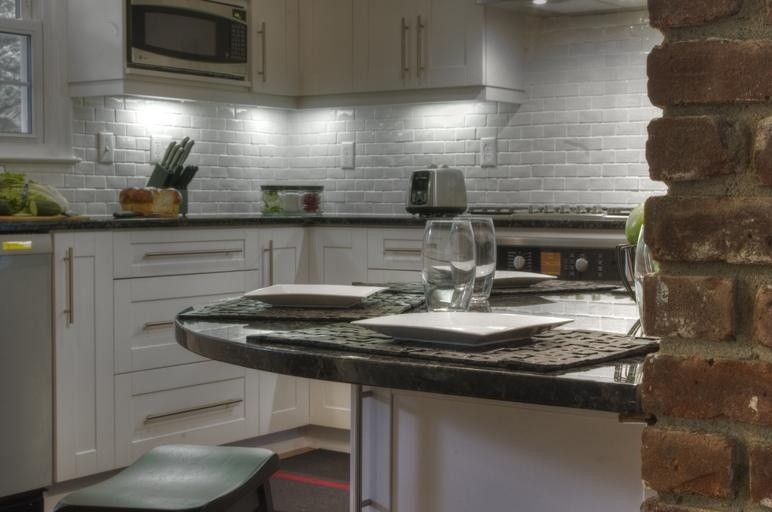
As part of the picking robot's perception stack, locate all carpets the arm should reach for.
[271,446,353,510]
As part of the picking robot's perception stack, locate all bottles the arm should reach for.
[260,185,324,216]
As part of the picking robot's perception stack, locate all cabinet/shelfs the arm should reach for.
[304,214,454,456]
[245,0,297,109]
[44,220,260,512]
[255,219,309,467]
[293,0,365,109]
[366,1,529,104]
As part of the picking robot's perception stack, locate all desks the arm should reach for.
[173,260,651,512]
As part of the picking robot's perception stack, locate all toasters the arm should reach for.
[405,163,467,218]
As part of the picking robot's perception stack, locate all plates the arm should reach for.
[352,312,574,349]
[244,284,389,308]
[430,263,557,287]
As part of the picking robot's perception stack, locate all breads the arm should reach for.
[118,186,183,218]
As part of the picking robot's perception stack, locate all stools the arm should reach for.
[42,442,277,510]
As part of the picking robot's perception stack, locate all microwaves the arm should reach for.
[126,0,249,82]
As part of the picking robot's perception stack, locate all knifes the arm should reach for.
[163,137,198,189]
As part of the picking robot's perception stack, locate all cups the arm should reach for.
[615,224,659,337]
[421,215,496,313]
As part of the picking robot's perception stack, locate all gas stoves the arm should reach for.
[470,204,641,281]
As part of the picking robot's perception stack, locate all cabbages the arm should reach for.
[0,171,70,214]
